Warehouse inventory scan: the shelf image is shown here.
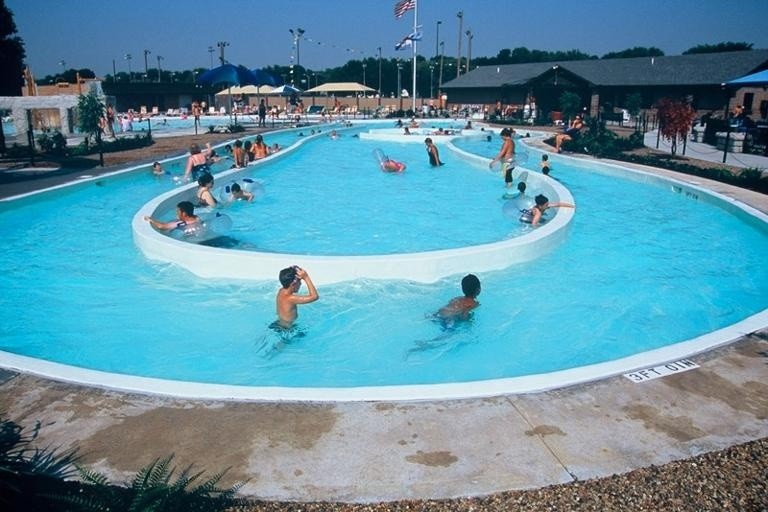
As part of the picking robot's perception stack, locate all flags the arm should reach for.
[395,31,423,49]
[394,0,416,21]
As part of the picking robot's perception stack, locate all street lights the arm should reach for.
[121,40,229,84]
[434,8,474,95]
[284,27,305,82]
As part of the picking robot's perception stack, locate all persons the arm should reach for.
[394,96,459,135]
[98,104,133,135]
[482,115,583,228]
[299,130,337,138]
[465,121,471,129]
[254,265,318,361]
[181,100,208,123]
[402,274,481,357]
[425,138,446,168]
[380,157,405,173]
[732,104,743,118]
[321,100,341,122]
[143,135,279,229]
[232,101,302,116]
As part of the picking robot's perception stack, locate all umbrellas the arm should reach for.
[270,82,302,114]
[239,68,284,120]
[197,61,256,125]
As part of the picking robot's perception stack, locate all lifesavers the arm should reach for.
[371,147,390,173]
[489,153,529,173]
[503,195,557,225]
[168,212,233,244]
[220,178,269,210]
[171,173,193,187]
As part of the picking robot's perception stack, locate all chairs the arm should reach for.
[125,105,159,121]
[166,107,189,119]
[329,106,360,115]
[205,106,226,117]
